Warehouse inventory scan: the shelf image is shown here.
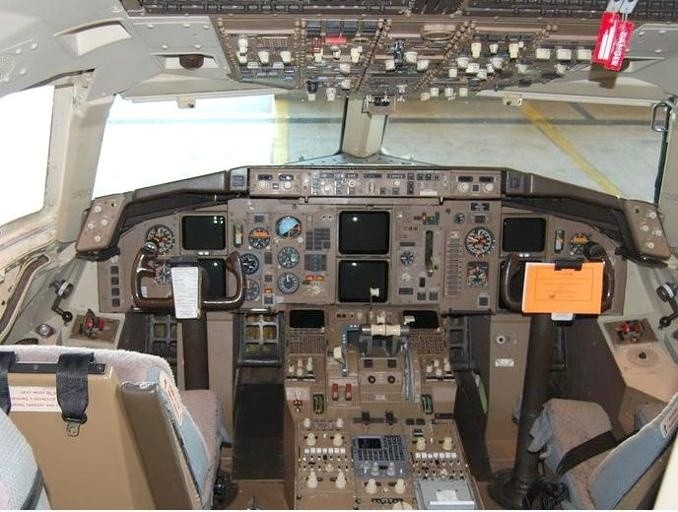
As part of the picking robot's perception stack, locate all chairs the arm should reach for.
[0,344,224,510]
[527,391,678,510]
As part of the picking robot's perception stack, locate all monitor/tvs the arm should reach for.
[502,217,546,253]
[337,209,389,256]
[196,259,225,300]
[179,213,226,251]
[338,259,389,303]
